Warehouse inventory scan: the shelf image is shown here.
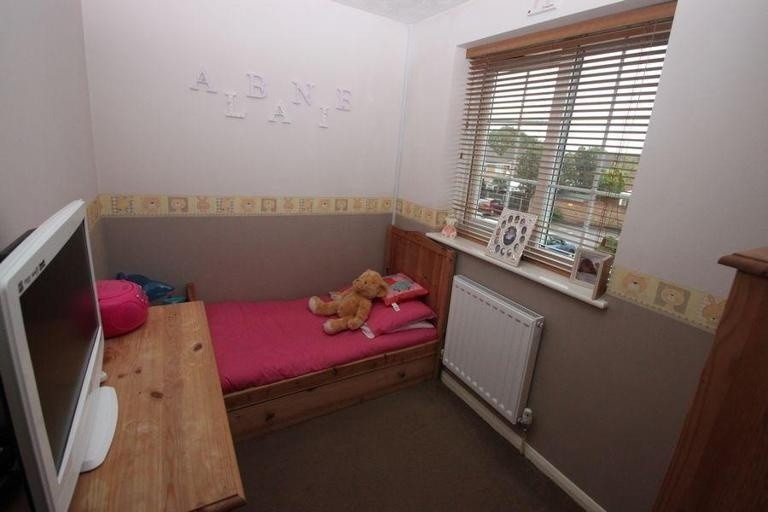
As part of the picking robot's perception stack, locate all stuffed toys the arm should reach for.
[308,267,389,335]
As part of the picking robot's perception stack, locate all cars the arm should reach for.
[619,190,631,198]
[543,231,577,258]
[475,175,528,217]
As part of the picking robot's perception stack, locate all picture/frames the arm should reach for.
[484,207,539,267]
[570,246,613,300]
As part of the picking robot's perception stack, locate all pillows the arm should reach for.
[329,276,436,341]
[96,279,149,338]
[378,272,429,306]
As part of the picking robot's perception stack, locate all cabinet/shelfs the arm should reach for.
[649,245,768,512]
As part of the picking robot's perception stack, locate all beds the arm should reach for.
[184,225,457,446]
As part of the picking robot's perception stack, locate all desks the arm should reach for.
[66,302,248,512]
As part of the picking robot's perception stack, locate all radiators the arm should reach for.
[438,273,545,429]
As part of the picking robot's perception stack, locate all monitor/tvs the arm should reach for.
[0,199,119,512]
